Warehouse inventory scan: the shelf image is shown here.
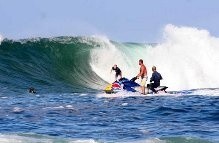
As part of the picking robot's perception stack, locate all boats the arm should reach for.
[121,75,168,94]
[103,77,129,94]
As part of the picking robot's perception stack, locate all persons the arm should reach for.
[112,65,122,81]
[149,66,162,94]
[137,59,147,95]
